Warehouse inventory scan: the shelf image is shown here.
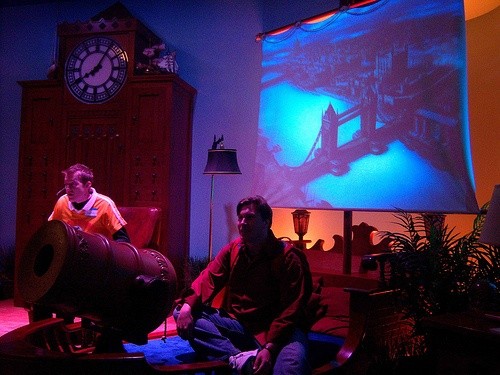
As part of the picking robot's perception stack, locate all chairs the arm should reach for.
[119,205,163,249]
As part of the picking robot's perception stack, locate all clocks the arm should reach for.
[64,36,127,105]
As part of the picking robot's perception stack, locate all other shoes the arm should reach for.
[229,349,259,374]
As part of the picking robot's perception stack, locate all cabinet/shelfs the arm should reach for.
[15,72,198,308]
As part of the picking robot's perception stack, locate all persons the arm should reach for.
[173,194,312,375]
[29,163,130,243]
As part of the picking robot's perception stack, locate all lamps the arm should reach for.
[283,210,311,258]
[199,134,242,263]
[479,185,500,312]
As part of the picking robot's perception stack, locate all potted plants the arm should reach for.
[368,199,500,311]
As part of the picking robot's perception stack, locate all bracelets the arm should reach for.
[264,344,276,356]
